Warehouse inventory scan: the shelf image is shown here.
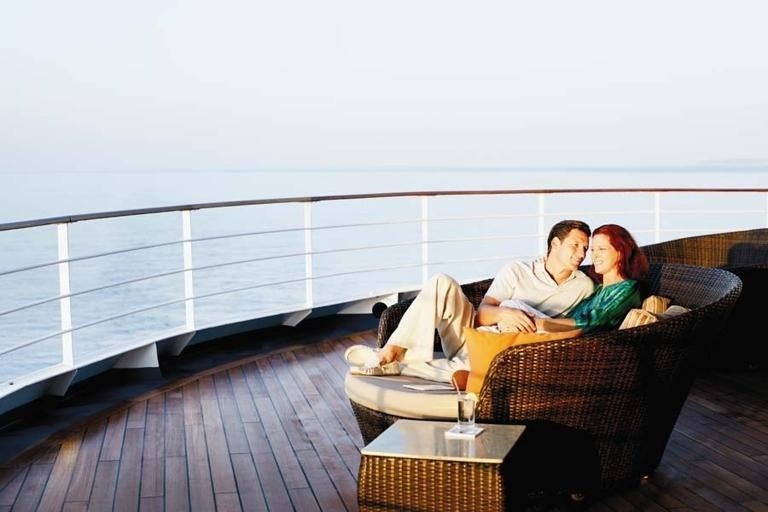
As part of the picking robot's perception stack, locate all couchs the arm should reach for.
[343,263,743,502]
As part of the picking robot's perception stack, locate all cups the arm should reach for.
[457,396,475,432]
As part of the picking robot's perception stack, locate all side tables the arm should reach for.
[356,419,526,511]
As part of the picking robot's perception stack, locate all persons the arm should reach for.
[476,224,650,333]
[344,220,596,384]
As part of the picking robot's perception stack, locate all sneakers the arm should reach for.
[344,344,381,366]
[349,355,401,375]
